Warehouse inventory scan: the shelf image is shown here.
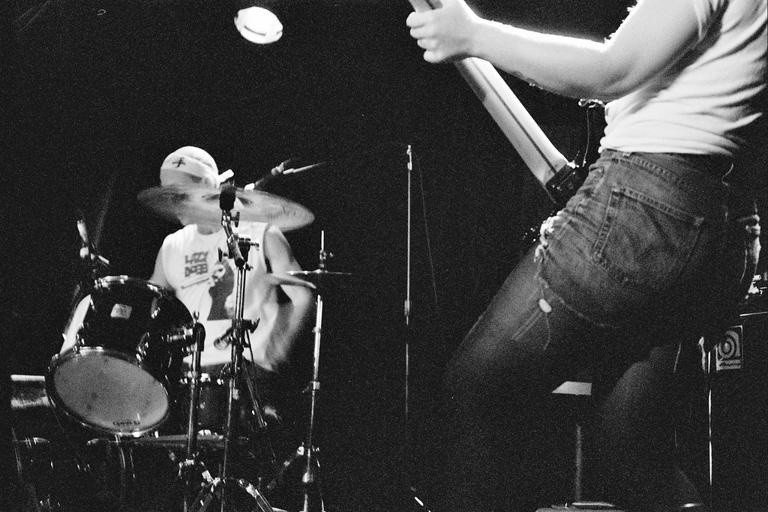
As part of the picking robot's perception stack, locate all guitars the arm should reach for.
[409,0,762,351]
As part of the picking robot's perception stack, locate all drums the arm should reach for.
[45,274,197,436]
[171,372,258,456]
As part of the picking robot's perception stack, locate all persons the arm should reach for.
[400,0,766,511]
[138,142,314,430]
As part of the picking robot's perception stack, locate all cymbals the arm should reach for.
[136,185,315,230]
[284,270,354,279]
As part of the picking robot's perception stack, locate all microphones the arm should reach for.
[241,155,295,193]
[79,245,111,267]
[219,182,247,268]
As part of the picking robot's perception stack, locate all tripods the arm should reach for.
[251,278,356,511]
[183,233,275,512]
